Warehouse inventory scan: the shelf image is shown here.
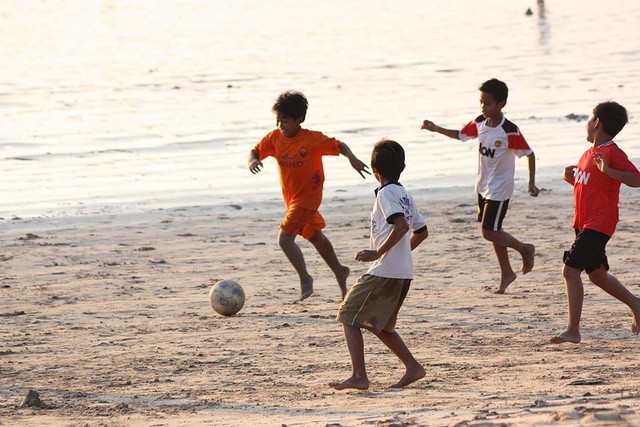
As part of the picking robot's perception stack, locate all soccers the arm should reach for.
[210,280,245,316]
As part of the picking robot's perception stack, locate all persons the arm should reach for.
[327,138,426,390]
[246,89,371,303]
[421,78,541,294]
[548,101,640,343]
